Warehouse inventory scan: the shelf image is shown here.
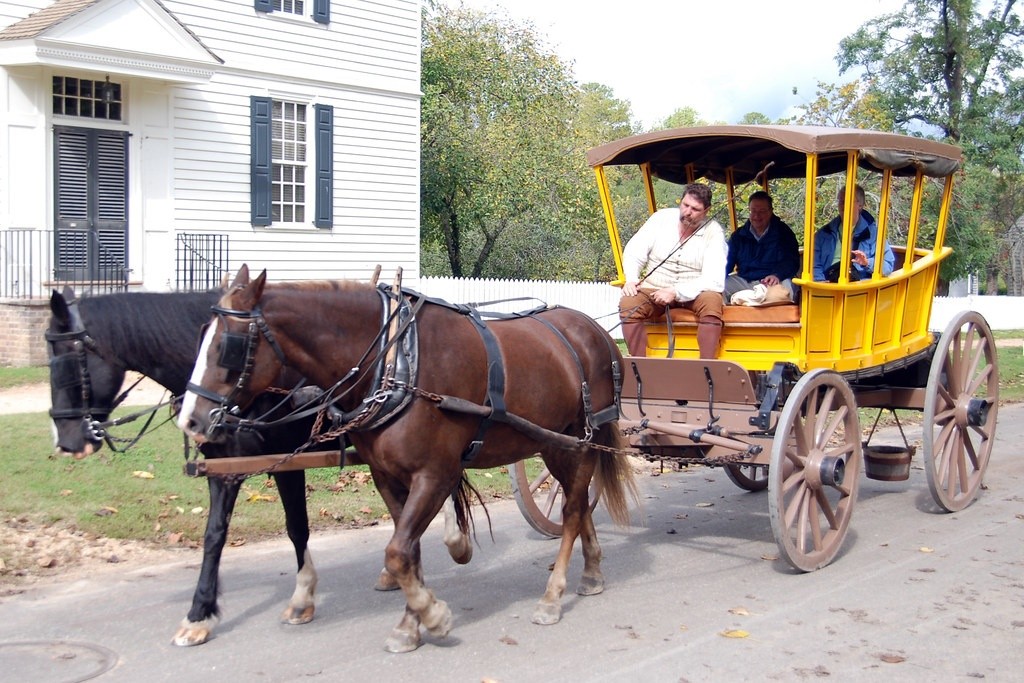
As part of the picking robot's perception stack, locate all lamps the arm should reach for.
[102,74,115,105]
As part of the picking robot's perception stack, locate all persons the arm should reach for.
[619,183,729,359]
[723,191,800,304]
[813,185,895,283]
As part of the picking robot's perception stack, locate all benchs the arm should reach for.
[642,302,799,323]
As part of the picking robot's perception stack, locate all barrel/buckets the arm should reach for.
[862,406,916,481]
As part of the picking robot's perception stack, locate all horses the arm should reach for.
[180,264,643,652]
[45,284,496,645]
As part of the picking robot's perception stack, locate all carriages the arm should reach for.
[44,126,1000,653]
